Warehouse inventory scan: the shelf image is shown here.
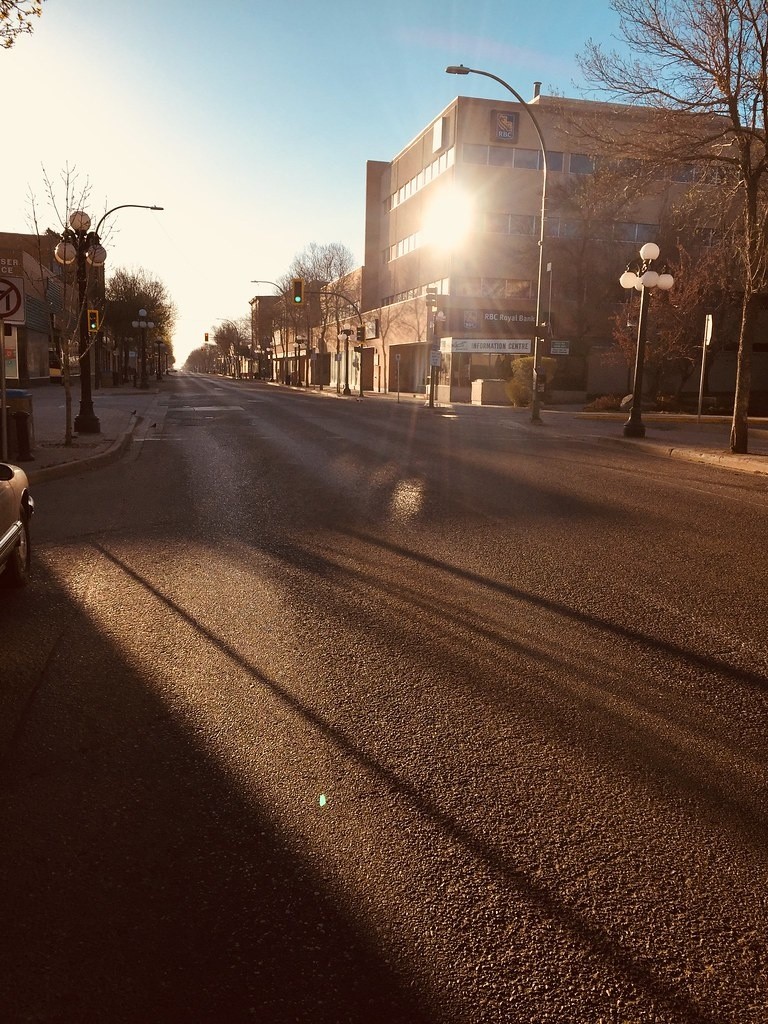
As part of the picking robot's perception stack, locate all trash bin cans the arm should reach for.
[0,389,34,463]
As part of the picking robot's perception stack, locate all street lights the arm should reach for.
[154,335,163,380]
[164,352,168,375]
[294,335,303,387]
[429,304,447,400]
[95,204,164,390]
[252,280,290,385]
[617,243,675,437]
[132,309,155,389]
[55,212,107,432]
[447,63,549,421]
[337,323,355,395]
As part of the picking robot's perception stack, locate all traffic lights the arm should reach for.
[426,287,438,308]
[291,278,305,305]
[356,325,365,344]
[534,322,549,350]
[86,309,99,332]
[205,333,208,341]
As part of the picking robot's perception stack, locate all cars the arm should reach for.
[0,462,35,593]
